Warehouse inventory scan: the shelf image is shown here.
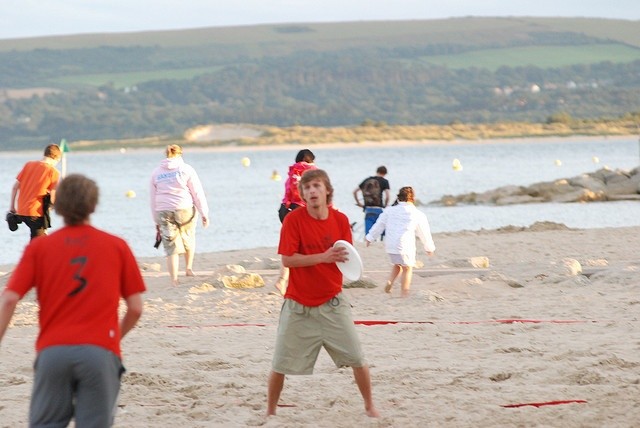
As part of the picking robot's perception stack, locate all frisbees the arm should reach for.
[333,240,362,282]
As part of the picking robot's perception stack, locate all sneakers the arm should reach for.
[6,214,18,231]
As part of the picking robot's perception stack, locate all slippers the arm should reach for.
[154,232,162,249]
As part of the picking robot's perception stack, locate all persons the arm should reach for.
[353,166,390,246]
[151,145,208,282]
[275,149,315,294]
[10,144,60,239]
[365,186,435,297]
[0,173,146,427]
[267,169,381,418]
